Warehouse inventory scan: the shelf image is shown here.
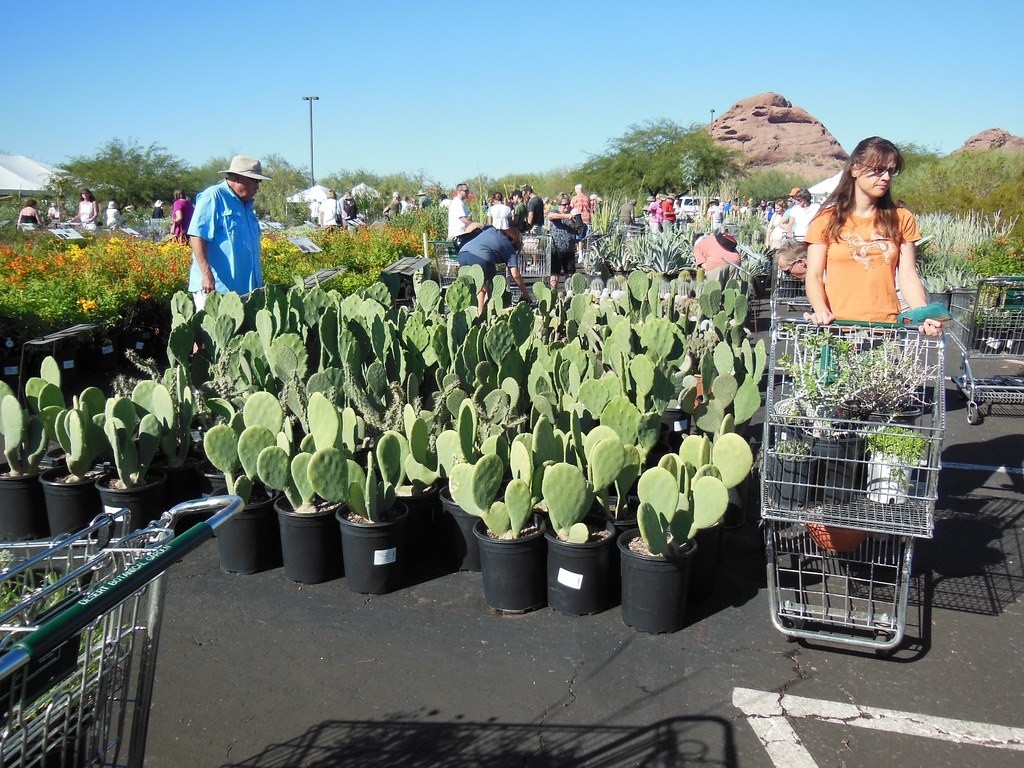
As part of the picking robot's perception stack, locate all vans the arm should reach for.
[675,195,720,220]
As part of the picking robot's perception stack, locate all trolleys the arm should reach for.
[946,275,1024,424]
[769,237,814,316]
[428,240,462,313]
[0,495,244,768]
[505,233,551,306]
[759,314,945,657]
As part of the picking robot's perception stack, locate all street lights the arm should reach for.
[303,96,319,187]
[710,109,715,136]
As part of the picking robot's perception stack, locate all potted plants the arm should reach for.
[0,231,1008,635]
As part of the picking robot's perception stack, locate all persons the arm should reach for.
[448,184,473,241]
[18,200,40,232]
[106,201,120,227]
[440,194,452,206]
[644,193,687,241]
[522,185,544,271]
[459,223,532,317]
[47,203,59,219]
[383,190,431,218]
[706,199,750,232]
[171,190,194,242]
[548,195,583,290]
[757,188,911,311]
[488,192,511,228]
[153,200,164,218]
[483,201,488,211]
[189,156,271,351]
[508,190,527,229]
[803,136,943,338]
[71,189,98,232]
[618,199,637,224]
[542,183,602,263]
[310,190,357,228]
[693,233,741,284]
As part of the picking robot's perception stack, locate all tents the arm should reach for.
[285,183,388,222]
[0,155,124,195]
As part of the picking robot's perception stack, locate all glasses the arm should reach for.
[857,161,900,176]
[784,261,797,275]
[560,201,570,206]
[236,175,262,183]
[796,197,804,201]
[460,190,468,192]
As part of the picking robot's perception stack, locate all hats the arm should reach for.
[691,233,703,242]
[509,190,522,199]
[707,199,718,206]
[218,156,273,180]
[656,194,665,200]
[646,196,655,201]
[155,200,163,207]
[667,194,676,201]
[107,201,115,208]
[345,196,354,200]
[415,190,426,196]
[50,202,58,206]
[787,188,800,196]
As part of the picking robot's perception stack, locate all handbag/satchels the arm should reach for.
[171,225,188,245]
[715,232,736,251]
[452,225,494,253]
[335,200,342,224]
